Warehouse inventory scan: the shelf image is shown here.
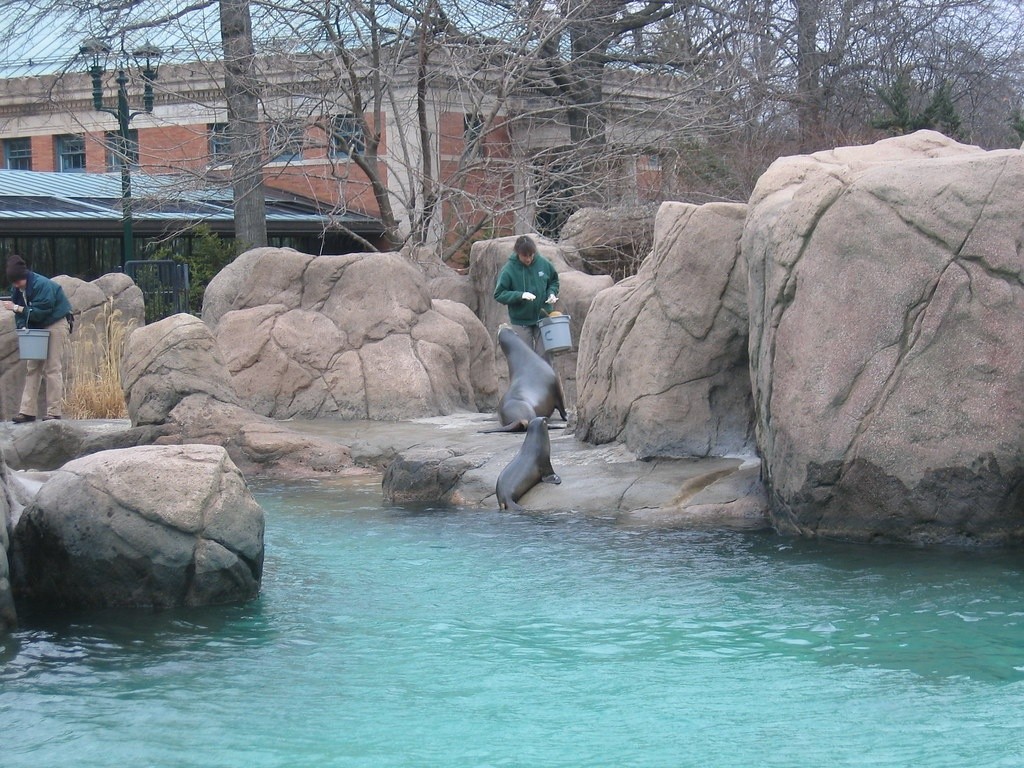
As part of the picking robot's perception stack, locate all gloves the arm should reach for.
[522,292,536,300]
[545,294,559,304]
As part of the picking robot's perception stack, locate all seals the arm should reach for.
[476,322,568,513]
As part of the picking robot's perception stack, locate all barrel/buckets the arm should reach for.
[536,297,573,353]
[15,308,50,360]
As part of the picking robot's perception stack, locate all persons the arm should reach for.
[493,236,560,366]
[2,255,72,423]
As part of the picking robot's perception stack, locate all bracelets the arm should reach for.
[14,305,19,312]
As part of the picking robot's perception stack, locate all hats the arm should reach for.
[6,255,27,282]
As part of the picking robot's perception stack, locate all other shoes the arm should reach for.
[42,415,61,421]
[12,414,37,422]
